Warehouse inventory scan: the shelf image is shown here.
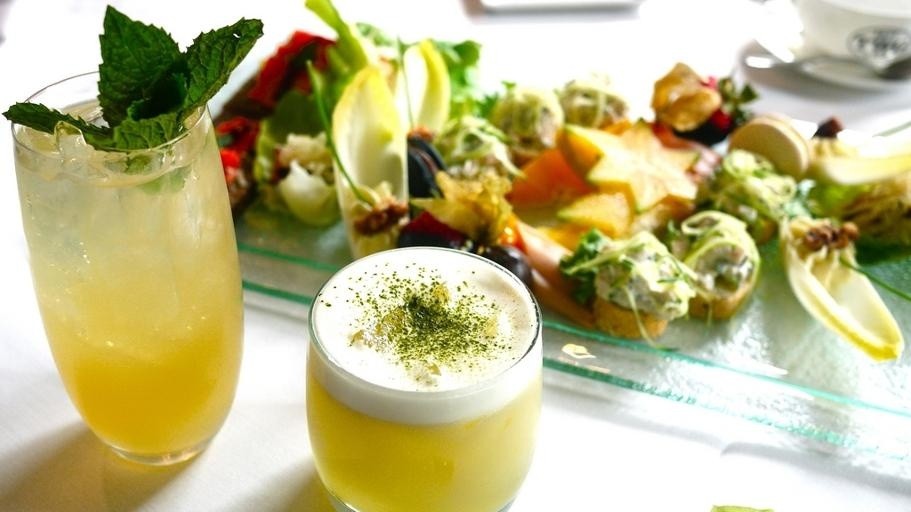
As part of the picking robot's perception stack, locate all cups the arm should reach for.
[11,70,245,469]
[304,247,545,512]
[804,1,911,63]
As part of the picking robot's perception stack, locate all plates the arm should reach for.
[751,1,911,95]
[237,128,911,458]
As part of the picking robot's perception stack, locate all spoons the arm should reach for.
[743,44,911,82]
